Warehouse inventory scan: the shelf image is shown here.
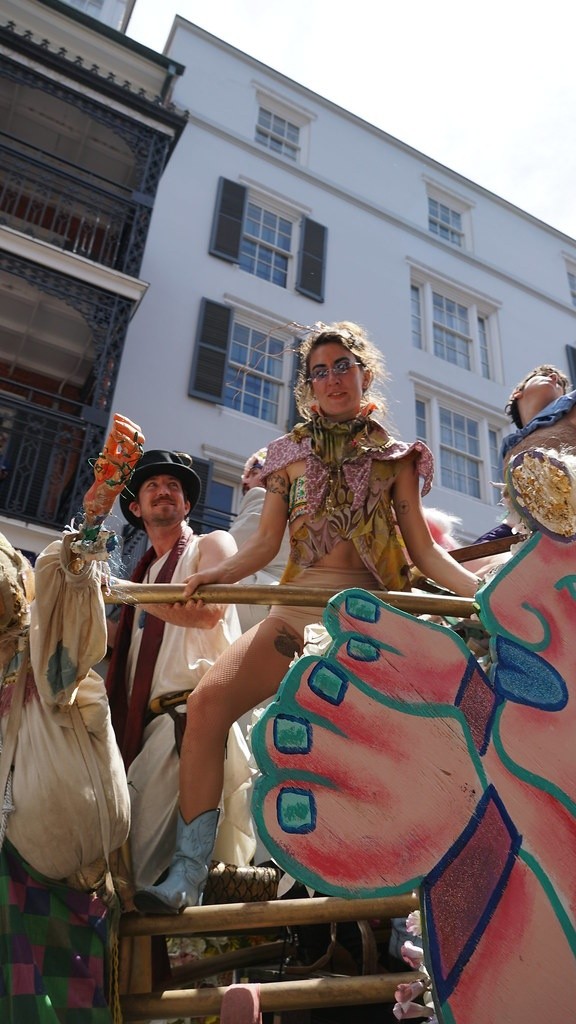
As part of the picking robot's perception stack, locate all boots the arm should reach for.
[134,808,222,915]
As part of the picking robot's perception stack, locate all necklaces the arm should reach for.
[138,539,173,630]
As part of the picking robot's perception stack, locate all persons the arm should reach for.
[132,320,488,922]
[472,363,576,546]
[102,449,261,902]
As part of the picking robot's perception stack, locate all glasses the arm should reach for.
[306,362,363,382]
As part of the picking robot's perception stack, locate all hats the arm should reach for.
[119,449,201,530]
